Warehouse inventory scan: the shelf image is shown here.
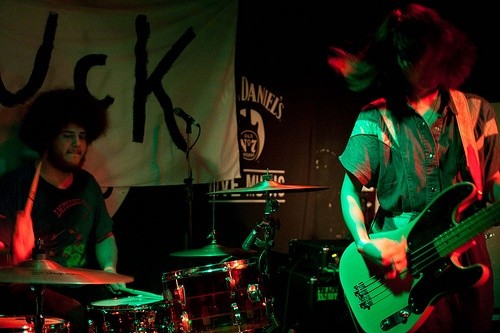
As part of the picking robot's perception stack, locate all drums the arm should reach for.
[161,257,267,333]
[87,293,163,333]
[0,314,71,333]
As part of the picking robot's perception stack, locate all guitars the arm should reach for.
[339,181,500,333]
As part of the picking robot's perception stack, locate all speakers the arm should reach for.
[286,271,365,333]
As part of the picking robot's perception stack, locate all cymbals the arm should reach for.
[170,244,259,257]
[0,254,135,286]
[205,180,330,194]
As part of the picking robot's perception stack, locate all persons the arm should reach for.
[0,87,126,317]
[327,4,500,333]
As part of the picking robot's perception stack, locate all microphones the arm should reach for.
[174,107,200,127]
